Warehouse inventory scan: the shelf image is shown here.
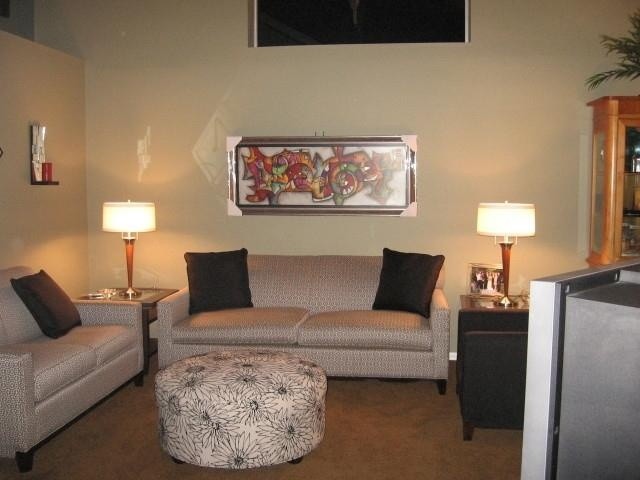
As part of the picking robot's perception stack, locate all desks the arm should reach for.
[73,285,178,378]
[461,294,531,312]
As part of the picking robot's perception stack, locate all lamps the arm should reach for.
[475,197,537,307]
[102,199,156,300]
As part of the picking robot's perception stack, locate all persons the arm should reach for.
[470,269,502,295]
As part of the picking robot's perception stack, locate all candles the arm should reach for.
[42,163,52,181]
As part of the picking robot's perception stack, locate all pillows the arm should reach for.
[368,247,447,319]
[11,270,83,338]
[179,248,255,315]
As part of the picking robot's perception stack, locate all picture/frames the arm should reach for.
[224,133,420,219]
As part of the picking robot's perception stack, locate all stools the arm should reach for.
[152,349,329,472]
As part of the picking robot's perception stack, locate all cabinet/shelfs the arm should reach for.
[584,95,640,269]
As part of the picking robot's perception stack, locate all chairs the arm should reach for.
[454,292,527,441]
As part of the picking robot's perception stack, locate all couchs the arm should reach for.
[0,261,150,473]
[152,247,455,399]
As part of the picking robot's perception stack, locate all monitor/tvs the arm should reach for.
[520,260,640,480]
